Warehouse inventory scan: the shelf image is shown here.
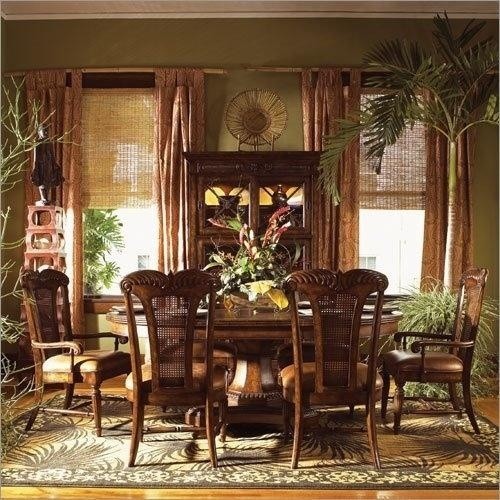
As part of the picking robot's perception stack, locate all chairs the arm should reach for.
[380,265,490,436]
[274,268,383,472]
[119,267,228,469]
[19,267,145,438]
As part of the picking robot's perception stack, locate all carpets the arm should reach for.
[0,392,500,489]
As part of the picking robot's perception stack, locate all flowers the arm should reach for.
[201,206,311,310]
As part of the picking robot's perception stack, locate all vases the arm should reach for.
[229,287,282,309]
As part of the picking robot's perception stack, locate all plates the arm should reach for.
[297,308,323,317]
[163,308,208,317]
[361,304,398,313]
[112,305,144,313]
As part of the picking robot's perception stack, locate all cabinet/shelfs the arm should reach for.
[182,148,341,297]
